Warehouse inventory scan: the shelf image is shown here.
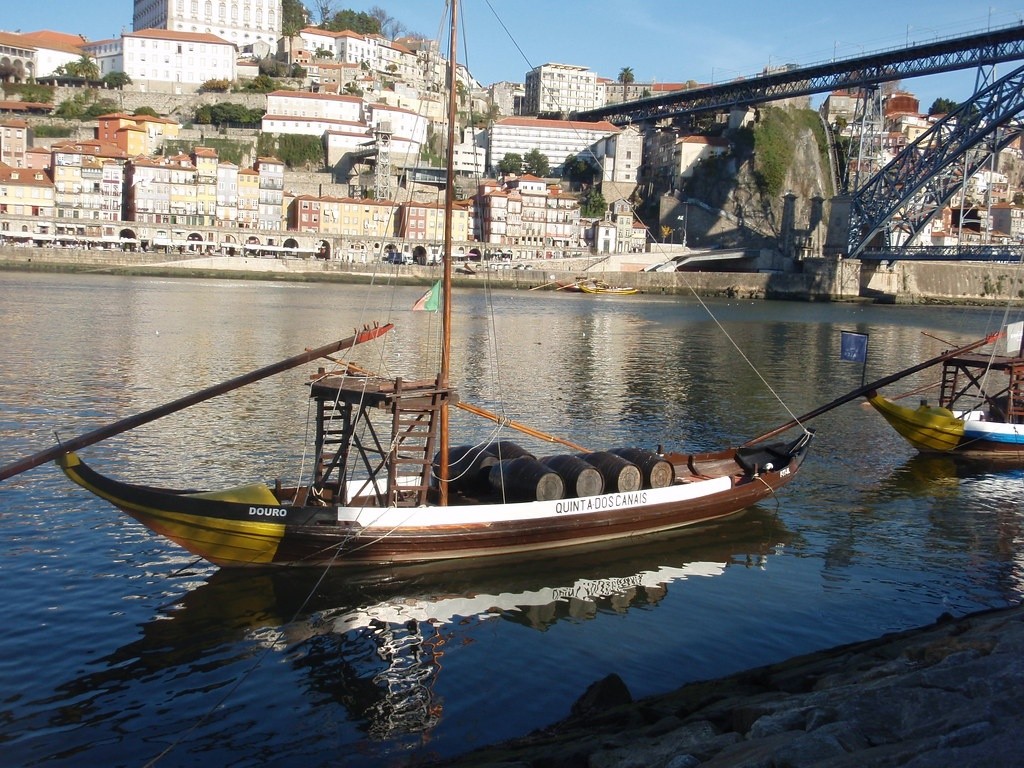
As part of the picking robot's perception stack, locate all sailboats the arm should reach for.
[45,0,821,573]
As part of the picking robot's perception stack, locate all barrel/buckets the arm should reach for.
[432,442,677,505]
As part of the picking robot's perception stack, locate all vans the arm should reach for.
[388,251,413,266]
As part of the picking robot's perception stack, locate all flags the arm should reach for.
[412,280,441,310]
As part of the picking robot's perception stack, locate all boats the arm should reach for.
[574,274,639,295]
[861,231,1024,456]
